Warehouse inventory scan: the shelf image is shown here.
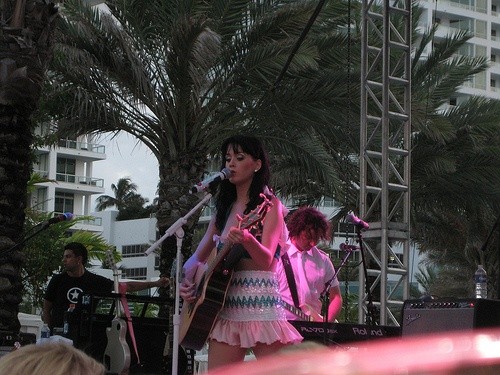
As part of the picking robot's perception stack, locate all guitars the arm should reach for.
[179,193,274,351]
[279,298,339,323]
[160,253,188,375]
[103,250,131,373]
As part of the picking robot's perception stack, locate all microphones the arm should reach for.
[188,168,232,194]
[346,215,369,229]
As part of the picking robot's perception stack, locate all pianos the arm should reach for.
[286,319,402,348]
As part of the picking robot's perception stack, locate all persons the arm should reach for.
[0,341,105,375]
[179,133,304,369]
[275,204,343,323]
[42,241,171,337]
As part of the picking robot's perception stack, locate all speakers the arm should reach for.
[399,298,500,336]
